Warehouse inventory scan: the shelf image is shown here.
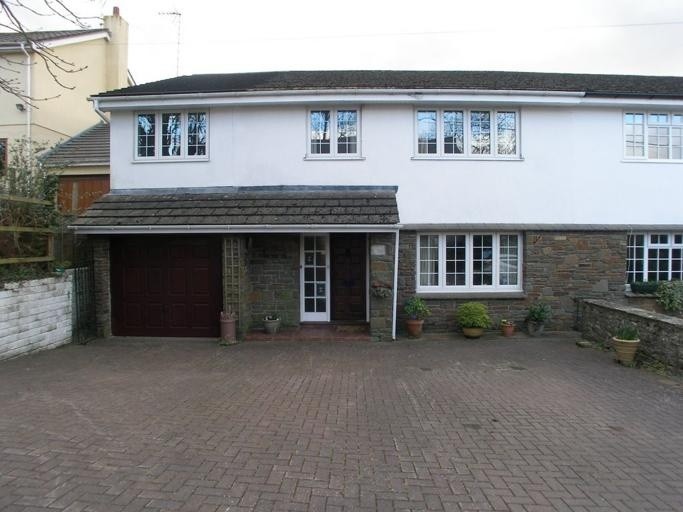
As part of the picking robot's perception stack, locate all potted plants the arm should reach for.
[262,313,281,335]
[611,325,641,365]
[525,302,550,336]
[404,296,432,337]
[456,301,490,339]
[501,320,515,336]
